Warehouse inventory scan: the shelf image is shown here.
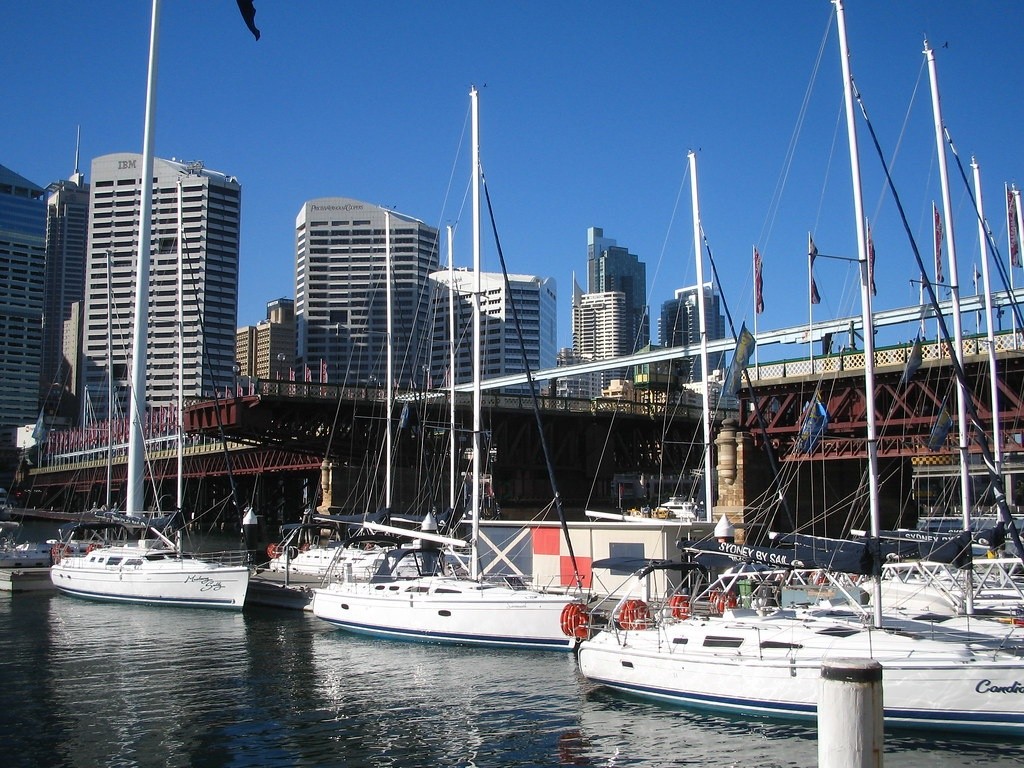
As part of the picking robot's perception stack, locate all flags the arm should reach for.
[722,186,1023,454]
[216,361,328,398]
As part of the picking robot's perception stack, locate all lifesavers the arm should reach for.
[86,543,99,554]
[670,595,693,620]
[561,602,592,638]
[266,541,283,560]
[709,589,737,615]
[619,600,650,631]
[808,570,828,587]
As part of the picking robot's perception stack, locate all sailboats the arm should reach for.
[0,1,1024,747]
